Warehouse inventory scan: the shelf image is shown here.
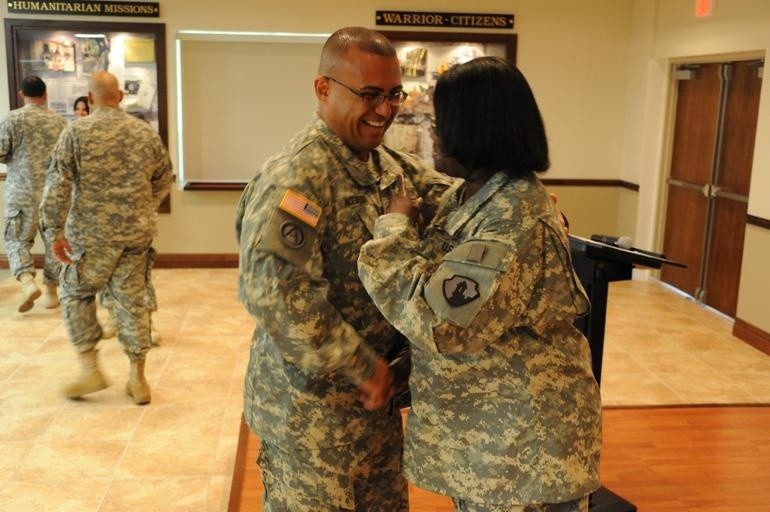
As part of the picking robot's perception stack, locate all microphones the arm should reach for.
[590,232,634,249]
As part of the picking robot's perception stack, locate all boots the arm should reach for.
[125,357,153,406]
[61,348,111,399]
[16,271,43,314]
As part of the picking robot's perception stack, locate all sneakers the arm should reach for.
[102,327,117,341]
[149,323,163,345]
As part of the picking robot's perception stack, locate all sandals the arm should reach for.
[44,283,61,309]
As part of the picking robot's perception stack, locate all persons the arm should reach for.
[35,70,173,405]
[232,26,443,511]
[355,55,603,512]
[96,247,161,347]
[0,75,66,311]
[73,96,89,118]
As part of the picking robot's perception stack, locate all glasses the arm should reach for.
[324,76,409,107]
